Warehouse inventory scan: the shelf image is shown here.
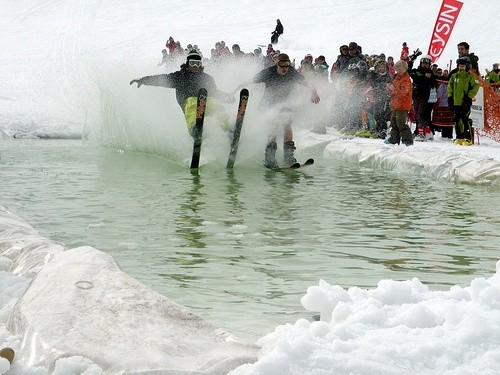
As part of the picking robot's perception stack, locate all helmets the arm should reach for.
[457,56,471,71]
[422,55,432,61]
[373,60,386,75]
[493,62,500,66]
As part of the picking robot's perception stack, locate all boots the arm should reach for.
[265,142,279,169]
[282,140,297,166]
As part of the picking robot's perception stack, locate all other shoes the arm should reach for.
[456,139,464,145]
[462,138,471,145]
[192,124,196,137]
[339,127,409,145]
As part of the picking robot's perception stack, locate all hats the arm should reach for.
[187,47,201,61]
[276,54,289,65]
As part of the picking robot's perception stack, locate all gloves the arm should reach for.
[447,96,454,108]
[463,97,472,106]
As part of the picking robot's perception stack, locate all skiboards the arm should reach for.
[262,158,314,169]
[226,88,249,168]
[190,88,208,168]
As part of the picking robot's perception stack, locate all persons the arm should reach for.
[270,19,283,44]
[447,57,480,146]
[129,48,236,141]
[484,63,500,92]
[232,53,321,169]
[383,60,413,146]
[156,36,481,141]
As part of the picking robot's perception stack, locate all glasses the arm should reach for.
[420,58,429,64]
[189,59,202,67]
[277,60,290,68]
[342,48,348,51]
[349,48,355,50]
